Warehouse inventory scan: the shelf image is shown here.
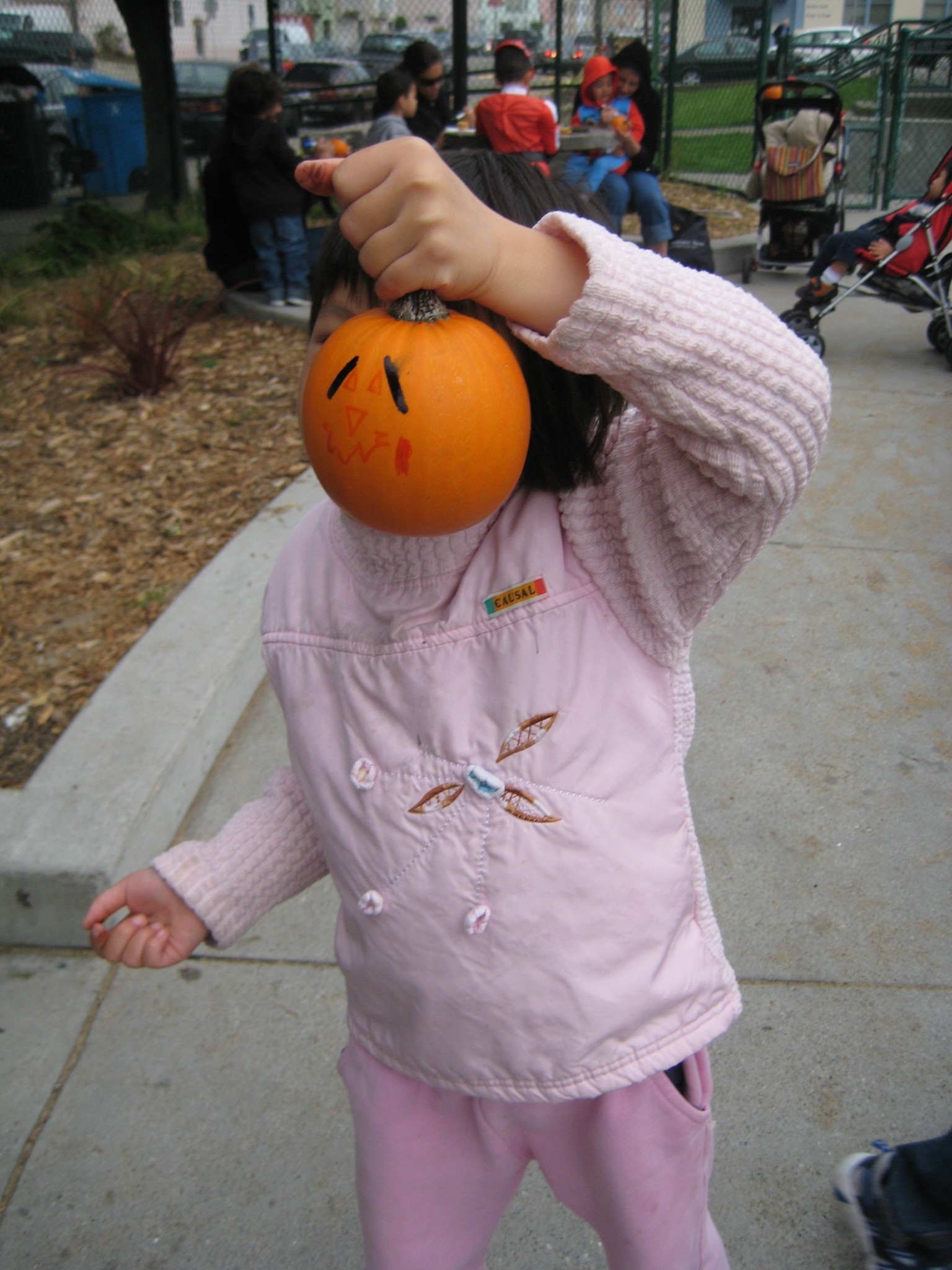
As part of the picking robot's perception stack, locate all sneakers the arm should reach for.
[832,1140,952,1270]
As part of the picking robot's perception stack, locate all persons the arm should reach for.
[795,161,952,306]
[219,68,316,311]
[200,135,344,300]
[829,1122,952,1270]
[74,131,833,1270]
[546,56,650,207]
[399,34,462,150]
[556,36,674,262]
[363,65,421,159]
[471,34,569,181]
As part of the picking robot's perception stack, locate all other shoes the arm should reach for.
[803,281,838,305]
[286,287,312,306]
[796,277,819,297]
[267,289,286,307]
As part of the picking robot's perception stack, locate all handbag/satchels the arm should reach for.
[667,205,714,274]
[758,145,825,202]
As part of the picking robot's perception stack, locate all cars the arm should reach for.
[527,29,670,78]
[272,59,378,124]
[240,29,500,82]
[0,13,97,203]
[763,26,879,73]
[172,57,245,147]
[658,33,805,88]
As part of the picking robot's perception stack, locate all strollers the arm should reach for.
[782,148,952,373]
[745,77,857,282]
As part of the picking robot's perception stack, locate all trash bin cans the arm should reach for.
[56,67,149,200]
[0,61,51,207]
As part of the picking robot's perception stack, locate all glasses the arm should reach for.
[417,75,443,87]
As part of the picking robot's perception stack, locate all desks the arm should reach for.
[444,127,616,174]
